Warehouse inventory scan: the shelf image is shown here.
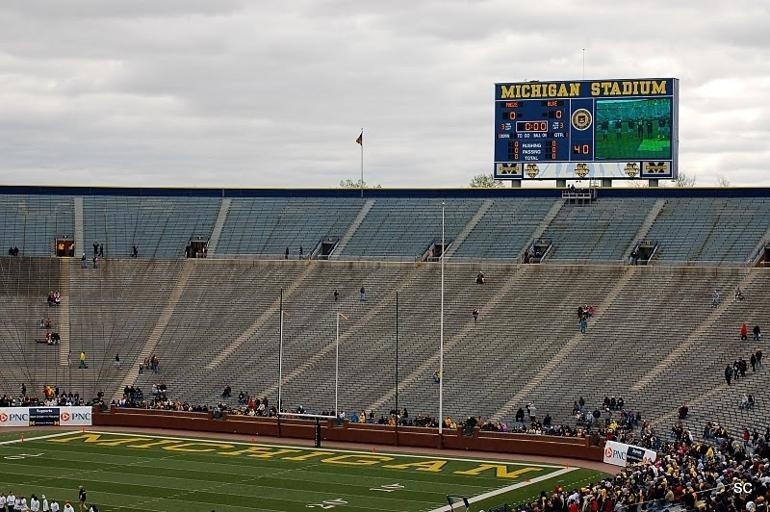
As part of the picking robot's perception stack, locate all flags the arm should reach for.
[356,135,362,145]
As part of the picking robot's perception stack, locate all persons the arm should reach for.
[58,242,65,256]
[0,351,770,512]
[334,289,340,300]
[68,242,74,257]
[81,240,103,269]
[567,182,576,192]
[601,116,671,141]
[47,288,61,306]
[740,323,749,340]
[735,287,743,300]
[45,331,60,344]
[524,245,543,263]
[40,317,51,329]
[360,285,366,299]
[629,249,640,265]
[473,308,479,320]
[284,247,289,260]
[754,322,760,340]
[578,303,593,332]
[185,245,207,258]
[298,246,304,260]
[712,290,720,304]
[476,270,485,282]
[9,246,19,256]
[133,244,139,256]
